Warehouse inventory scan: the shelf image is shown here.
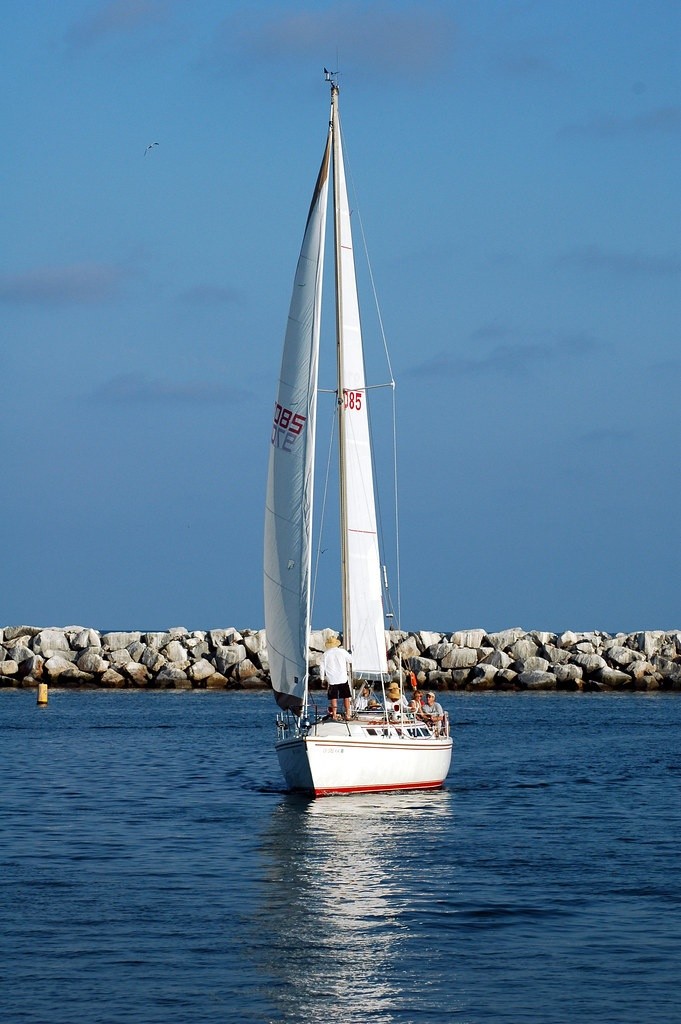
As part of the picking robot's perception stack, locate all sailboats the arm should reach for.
[251,65,458,803]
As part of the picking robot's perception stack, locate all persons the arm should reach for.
[320,635,353,721]
[320,682,442,735]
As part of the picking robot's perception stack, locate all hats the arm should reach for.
[388,683,400,690]
[325,636,341,648]
[426,692,435,698]
[387,689,401,699]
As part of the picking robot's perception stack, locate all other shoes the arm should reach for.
[333,717,338,720]
[346,715,353,721]
